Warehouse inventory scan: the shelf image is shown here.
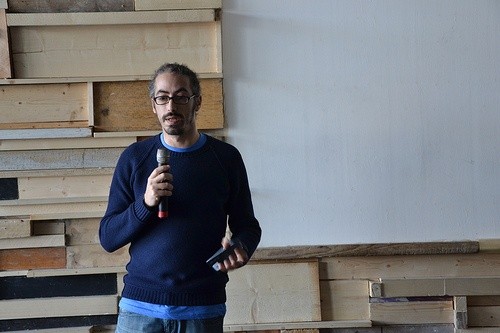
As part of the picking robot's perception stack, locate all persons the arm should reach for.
[97,63,262,332]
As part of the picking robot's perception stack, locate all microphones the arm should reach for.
[157,150,169,219]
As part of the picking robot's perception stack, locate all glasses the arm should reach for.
[151,94,198,105]
